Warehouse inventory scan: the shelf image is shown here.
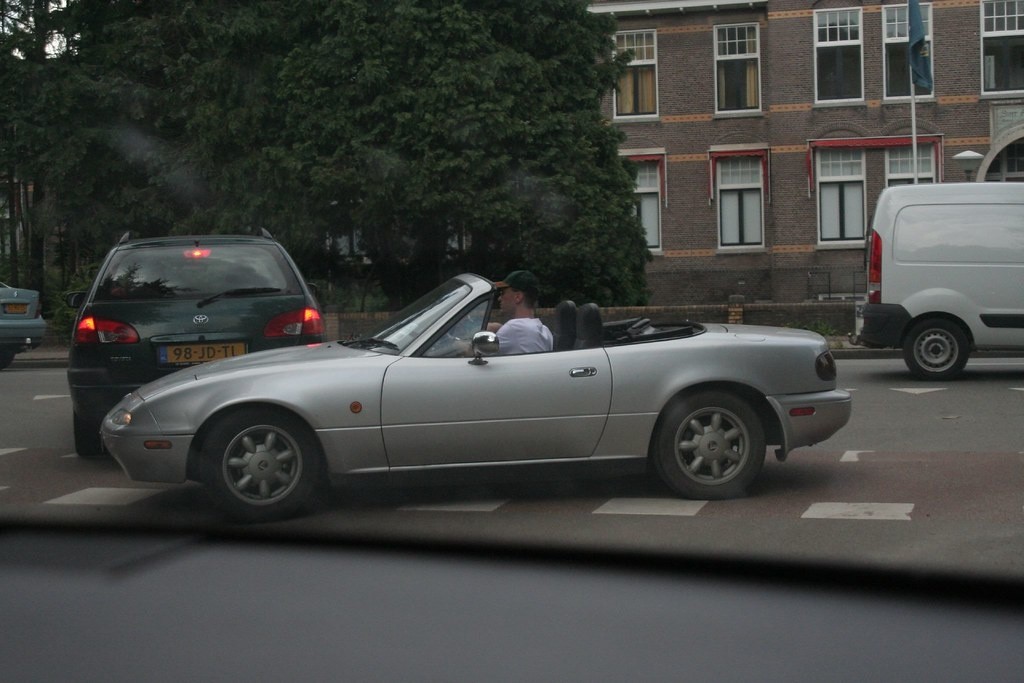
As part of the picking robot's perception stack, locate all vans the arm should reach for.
[855,182,1024,381]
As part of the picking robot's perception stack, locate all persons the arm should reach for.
[457,269,553,356]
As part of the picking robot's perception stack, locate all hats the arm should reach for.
[496,271,540,294]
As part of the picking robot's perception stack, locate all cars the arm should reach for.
[67,231,324,457]
[96,272,852,525]
[0,283,47,372]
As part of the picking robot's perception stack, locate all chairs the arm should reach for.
[554,301,577,350]
[574,303,604,349]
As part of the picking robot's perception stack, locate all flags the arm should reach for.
[907,0,933,91]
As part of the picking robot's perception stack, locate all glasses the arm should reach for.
[500,290,528,298]
